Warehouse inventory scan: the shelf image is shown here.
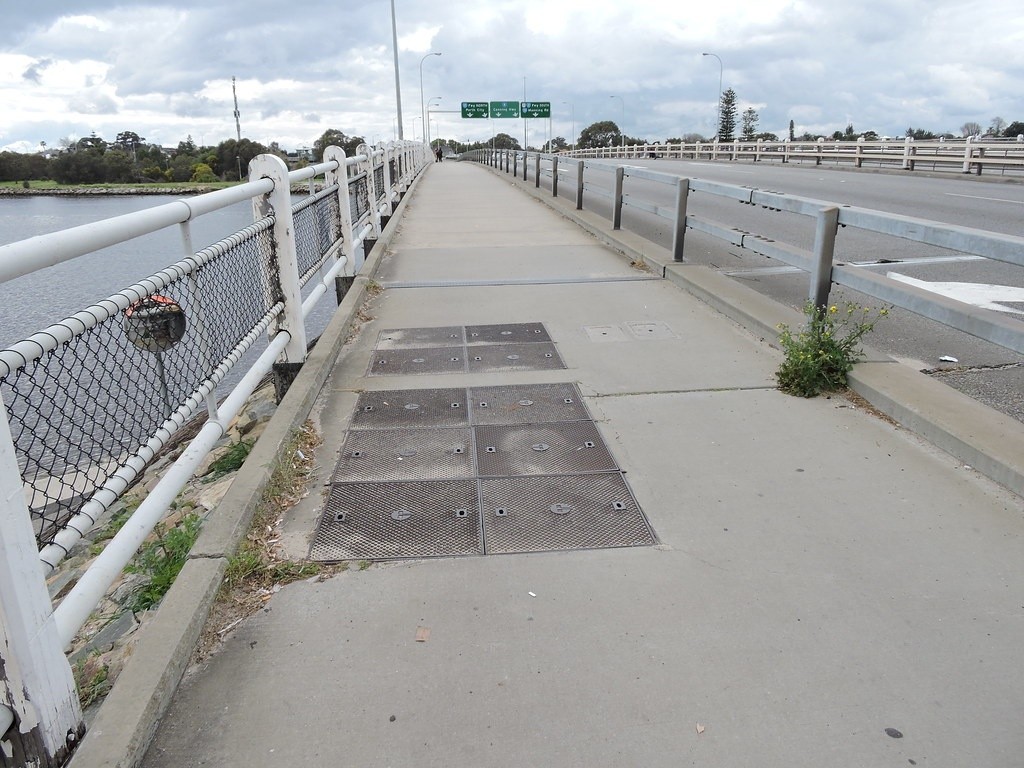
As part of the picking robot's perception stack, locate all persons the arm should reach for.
[436,147,443,162]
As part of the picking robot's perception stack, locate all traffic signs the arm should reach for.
[520,102,551,118]
[489,101,520,118]
[461,102,489,119]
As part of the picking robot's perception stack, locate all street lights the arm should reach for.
[703,52,722,139]
[236,156,242,180]
[610,95,625,146]
[420,53,442,142]
[413,117,422,141]
[562,101,575,151]
[427,97,442,144]
[425,95,441,142]
